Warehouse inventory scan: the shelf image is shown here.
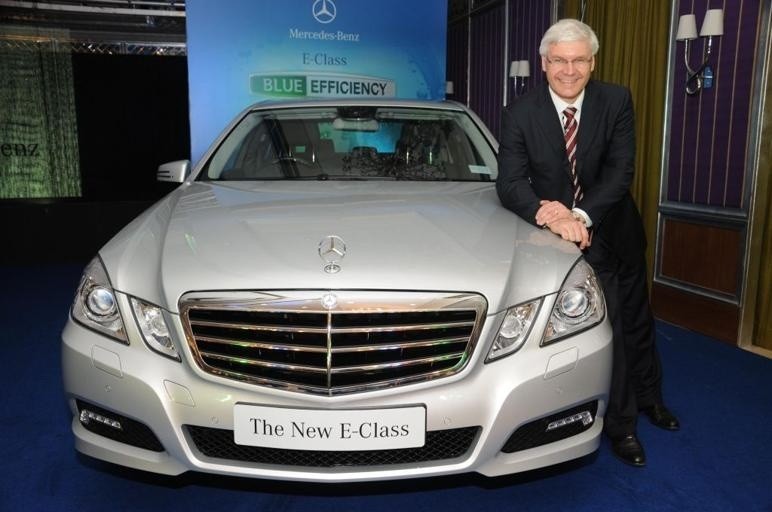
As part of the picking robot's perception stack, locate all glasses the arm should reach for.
[545,55,593,68]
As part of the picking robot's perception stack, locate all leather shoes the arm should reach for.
[607,432,647,467]
[638,400,681,431]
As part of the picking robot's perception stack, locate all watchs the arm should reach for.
[570,210,586,224]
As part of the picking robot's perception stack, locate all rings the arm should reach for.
[554,209,559,215]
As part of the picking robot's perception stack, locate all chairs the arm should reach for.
[254,120,452,181]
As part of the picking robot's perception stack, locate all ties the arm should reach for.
[561,107,584,206]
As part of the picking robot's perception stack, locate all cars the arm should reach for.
[57,98,617,485]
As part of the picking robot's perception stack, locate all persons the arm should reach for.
[496,18,681,468]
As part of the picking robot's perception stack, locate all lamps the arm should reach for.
[509,60,530,98]
[675,8,727,96]
